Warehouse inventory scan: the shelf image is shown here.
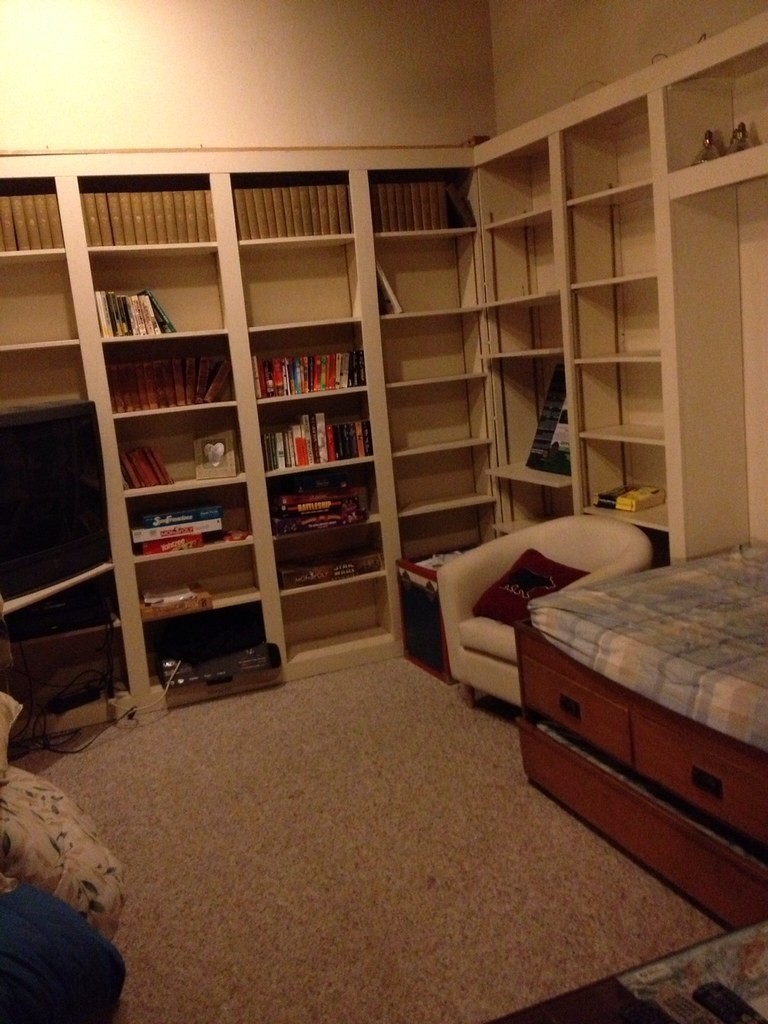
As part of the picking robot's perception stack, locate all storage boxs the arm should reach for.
[395,543,482,686]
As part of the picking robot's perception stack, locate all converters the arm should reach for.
[47,686,101,714]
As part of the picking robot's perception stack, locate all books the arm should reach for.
[118,446,176,489]
[592,483,666,512]
[261,412,373,472]
[250,349,366,399]
[95,288,177,337]
[105,355,231,413]
[0,180,477,253]
[375,256,403,314]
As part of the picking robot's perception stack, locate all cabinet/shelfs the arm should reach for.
[0,6,768,739]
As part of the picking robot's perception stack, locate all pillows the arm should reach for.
[472,546,591,626]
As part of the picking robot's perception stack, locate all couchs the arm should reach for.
[435,513,654,705]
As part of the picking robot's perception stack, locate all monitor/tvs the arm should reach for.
[0,400,112,600]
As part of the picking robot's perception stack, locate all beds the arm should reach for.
[514,544,768,933]
